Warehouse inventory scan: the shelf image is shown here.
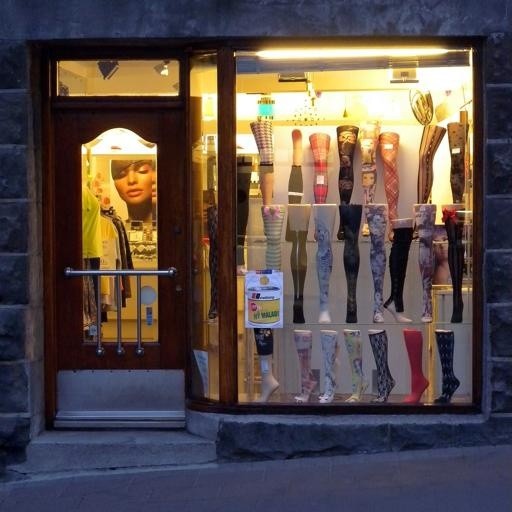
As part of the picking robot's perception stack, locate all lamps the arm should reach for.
[154,63,168,76]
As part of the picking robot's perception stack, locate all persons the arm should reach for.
[151,161,157,225]
[111,159,152,229]
[207,203,220,320]
[237,121,469,403]
